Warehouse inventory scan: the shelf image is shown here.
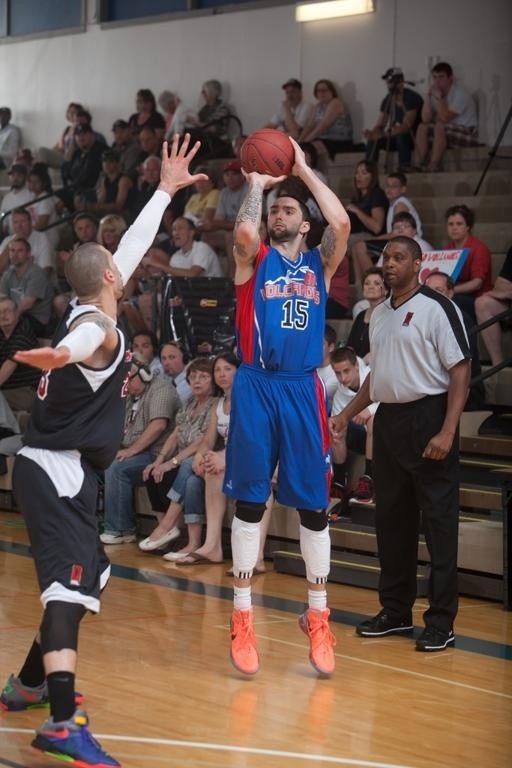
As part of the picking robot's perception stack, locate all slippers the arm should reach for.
[175,552,226,566]
[225,566,270,576]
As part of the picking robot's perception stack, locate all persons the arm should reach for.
[2,62,512,576]
[218,137,350,677]
[329,235,472,650]
[2,128,209,768]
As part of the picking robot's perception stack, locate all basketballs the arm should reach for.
[240,129,294,178]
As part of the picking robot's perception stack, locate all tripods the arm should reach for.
[369,82,425,173]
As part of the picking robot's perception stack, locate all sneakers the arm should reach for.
[415,626,455,651]
[349,474,377,505]
[0,672,84,711]
[100,526,190,562]
[298,607,337,675]
[230,606,260,674]
[356,607,414,638]
[31,710,121,768]
[329,475,347,505]
[396,161,444,174]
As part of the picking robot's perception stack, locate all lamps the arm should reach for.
[294,0,375,22]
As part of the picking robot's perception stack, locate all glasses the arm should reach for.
[384,184,403,189]
[393,224,410,229]
[187,375,210,382]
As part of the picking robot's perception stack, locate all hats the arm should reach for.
[112,120,126,131]
[8,164,27,174]
[222,161,242,173]
[283,78,301,89]
[75,123,91,134]
[16,149,32,160]
